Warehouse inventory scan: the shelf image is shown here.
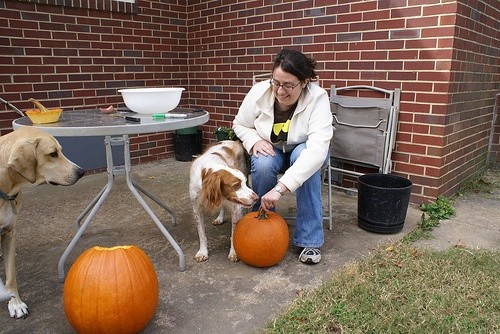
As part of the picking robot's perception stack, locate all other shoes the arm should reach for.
[297,246,324,264]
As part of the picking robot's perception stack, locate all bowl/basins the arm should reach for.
[25,108,63,124]
[118,87,185,118]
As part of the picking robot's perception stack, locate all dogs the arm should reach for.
[188,140,260,264]
[0,126,85,320]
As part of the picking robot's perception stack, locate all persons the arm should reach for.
[232,49,333,263]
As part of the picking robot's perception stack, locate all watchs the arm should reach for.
[275,185,284,196]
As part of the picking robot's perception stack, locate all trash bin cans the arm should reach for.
[172,130,203,162]
[358,174,413,234]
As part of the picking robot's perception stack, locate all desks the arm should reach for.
[12,106,210,280]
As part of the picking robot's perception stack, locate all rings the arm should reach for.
[270,204,272,206]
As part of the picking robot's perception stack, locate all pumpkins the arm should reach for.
[24,98,63,123]
[63,244,158,334]
[232,205,289,267]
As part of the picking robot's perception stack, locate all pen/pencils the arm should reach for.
[126,117,140,122]
[153,114,188,117]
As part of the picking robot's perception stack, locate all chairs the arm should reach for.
[245,74,332,231]
[328,85,401,193]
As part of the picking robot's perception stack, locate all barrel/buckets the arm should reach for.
[358,174,417,237]
[171,129,202,162]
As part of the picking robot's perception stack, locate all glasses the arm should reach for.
[270,73,303,92]
